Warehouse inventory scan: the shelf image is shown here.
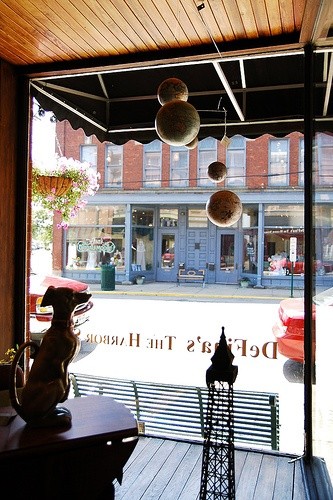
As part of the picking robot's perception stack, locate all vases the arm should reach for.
[32,176,71,199]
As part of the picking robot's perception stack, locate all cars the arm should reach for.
[29,269,93,331]
[163,247,175,269]
[269,252,333,277]
[279,286,333,367]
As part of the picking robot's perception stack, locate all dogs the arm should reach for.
[8,285,94,432]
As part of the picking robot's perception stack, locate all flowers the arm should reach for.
[31,153,99,228]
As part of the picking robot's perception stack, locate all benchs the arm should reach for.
[176,268,207,286]
[67,374,282,452]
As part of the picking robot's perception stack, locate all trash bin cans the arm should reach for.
[101,265,116,291]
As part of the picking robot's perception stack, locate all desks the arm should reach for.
[0,394,140,500]
